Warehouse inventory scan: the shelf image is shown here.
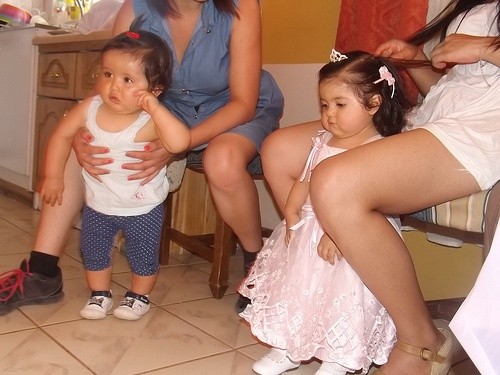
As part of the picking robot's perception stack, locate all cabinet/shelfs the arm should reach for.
[32,32,222,259]
[0,27,57,204]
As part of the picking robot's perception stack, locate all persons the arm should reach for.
[1,1,284,313]
[261,0,500,375]
[238,49,433,375]
[39,15,191,320]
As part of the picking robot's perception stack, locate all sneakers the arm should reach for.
[113,292,150,320]
[0,261,65,316]
[78,290,114,320]
[236,261,260,313]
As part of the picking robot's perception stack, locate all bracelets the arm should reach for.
[479,36,488,67]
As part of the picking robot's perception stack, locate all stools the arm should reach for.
[387,175,500,266]
[155,158,285,300]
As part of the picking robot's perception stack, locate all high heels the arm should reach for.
[374,329,451,375]
